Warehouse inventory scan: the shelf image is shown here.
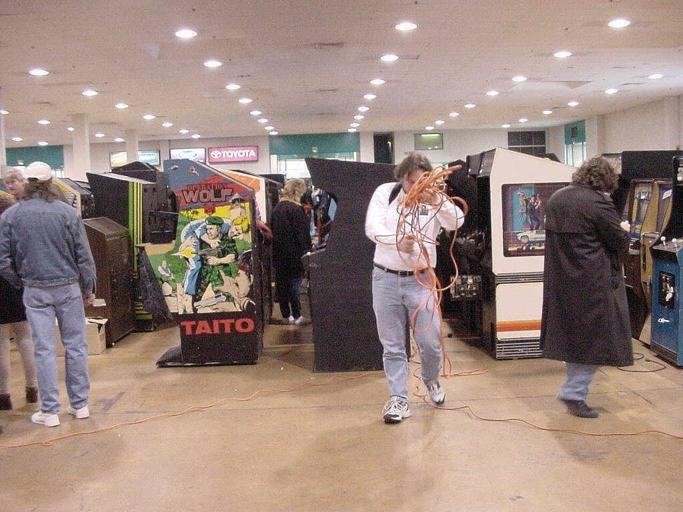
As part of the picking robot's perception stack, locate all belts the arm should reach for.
[374,263,434,277]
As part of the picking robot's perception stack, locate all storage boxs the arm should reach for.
[53,316,108,356]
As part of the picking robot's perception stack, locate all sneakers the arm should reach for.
[428,383,446,405]
[294,316,313,326]
[67,405,90,419]
[382,399,412,423]
[25,387,38,403]
[557,395,598,418]
[31,410,60,427]
[0,394,13,410]
[282,315,294,325]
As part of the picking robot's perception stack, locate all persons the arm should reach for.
[0,192,38,410]
[539,157,631,417]
[448,159,479,326]
[179,216,241,313]
[255,219,274,241]
[271,178,313,326]
[4,167,29,203]
[364,150,464,422]
[520,192,544,230]
[0,161,98,427]
[299,191,333,245]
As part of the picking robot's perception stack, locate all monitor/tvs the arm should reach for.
[632,190,650,230]
[654,184,674,234]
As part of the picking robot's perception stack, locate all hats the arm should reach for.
[25,161,52,181]
